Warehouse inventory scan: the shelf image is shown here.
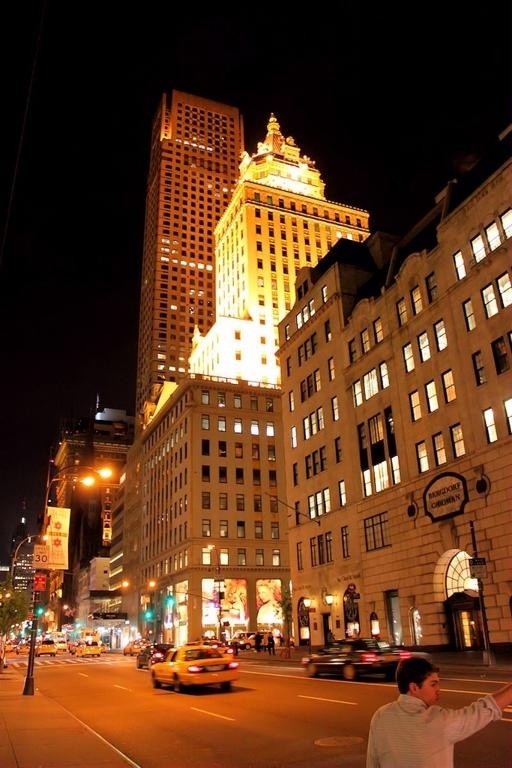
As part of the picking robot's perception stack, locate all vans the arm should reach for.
[228,631,275,651]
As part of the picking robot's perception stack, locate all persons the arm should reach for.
[328,633,335,642]
[268,632,276,656]
[366,656,512,768]
[238,633,247,656]
[352,629,358,639]
[253,632,263,653]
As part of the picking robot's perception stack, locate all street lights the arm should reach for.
[20,445,115,696]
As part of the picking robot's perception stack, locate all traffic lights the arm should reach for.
[145,610,154,622]
[166,593,177,608]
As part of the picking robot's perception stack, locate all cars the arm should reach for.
[136,643,175,670]
[299,638,410,683]
[148,642,244,696]
[123,638,153,657]
[6,626,111,660]
